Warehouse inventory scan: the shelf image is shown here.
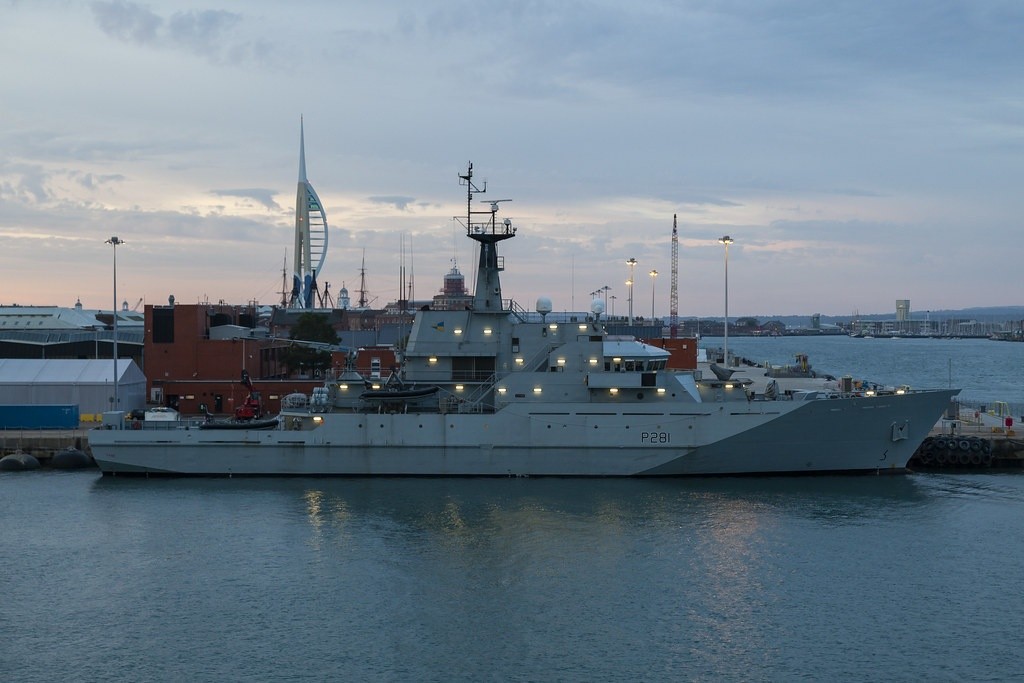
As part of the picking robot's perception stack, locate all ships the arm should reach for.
[84,155,964,481]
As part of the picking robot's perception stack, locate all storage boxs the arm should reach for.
[0,404,80,430]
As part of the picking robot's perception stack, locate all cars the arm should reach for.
[131,408,154,421]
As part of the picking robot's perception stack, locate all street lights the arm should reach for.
[650,270,659,326]
[105,235,126,412]
[626,257,636,327]
[590,286,618,335]
[717,235,733,371]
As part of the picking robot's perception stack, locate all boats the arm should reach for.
[197,402,279,429]
[359,378,440,404]
